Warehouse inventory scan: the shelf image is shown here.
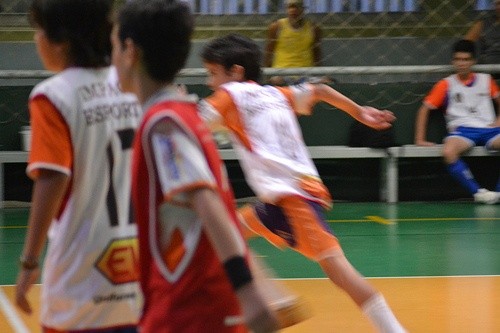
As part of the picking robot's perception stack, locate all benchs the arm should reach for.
[0,145,500,203]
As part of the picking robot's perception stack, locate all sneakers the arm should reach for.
[473,188,500,204]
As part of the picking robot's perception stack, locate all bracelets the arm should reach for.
[18,255,39,269]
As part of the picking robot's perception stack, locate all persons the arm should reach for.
[110,0,282,333]
[264,0,321,85]
[173,34,411,333]
[16,0,145,333]
[414,39,500,204]
